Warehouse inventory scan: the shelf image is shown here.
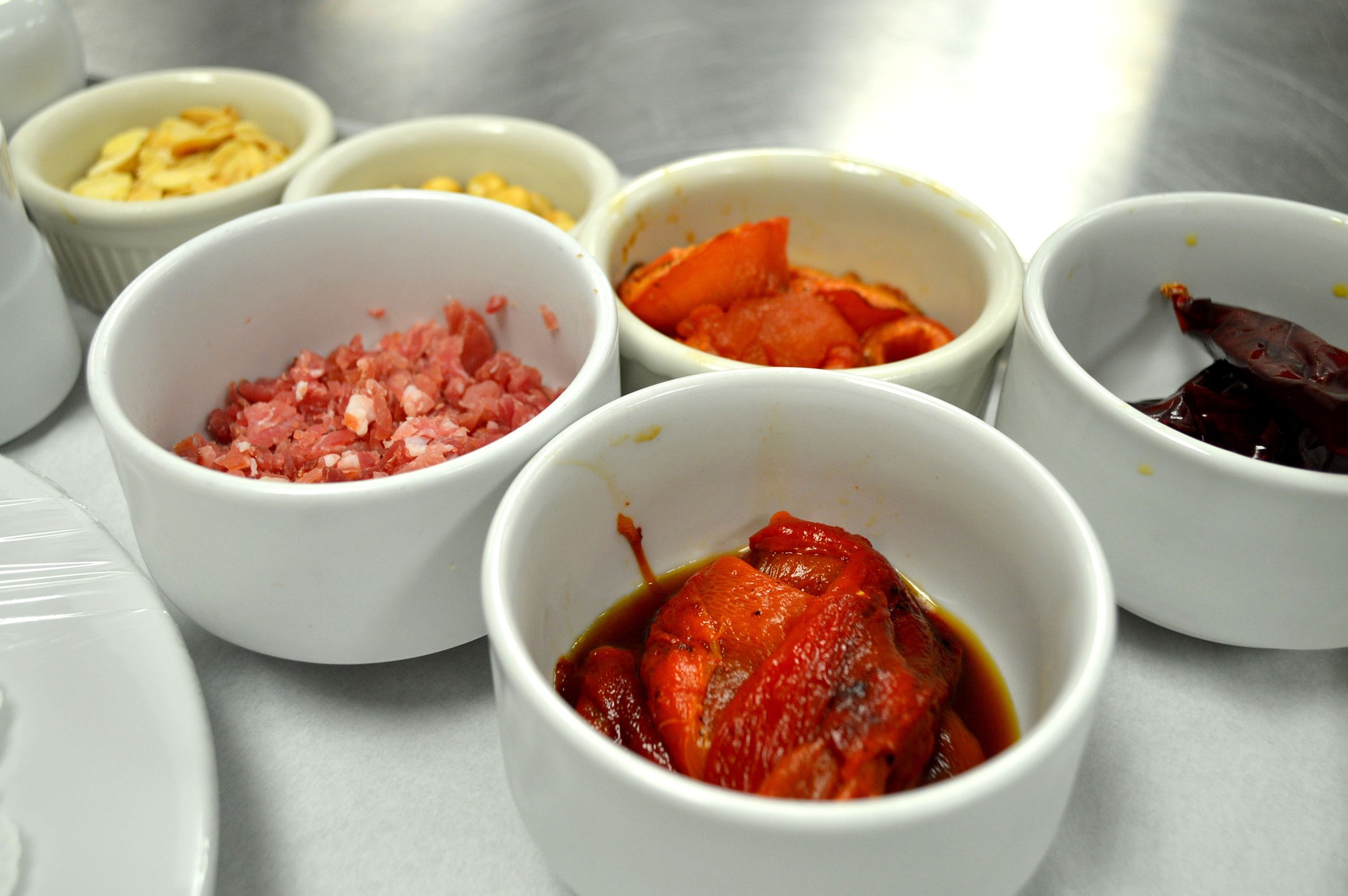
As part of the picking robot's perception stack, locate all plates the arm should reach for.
[1,454,220,896]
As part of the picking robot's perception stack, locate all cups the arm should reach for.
[1,0,87,447]
[579,149,1025,420]
[278,114,618,242]
[479,368,1118,894]
[995,190,1348,651]
[85,187,623,667]
[8,67,337,313]
